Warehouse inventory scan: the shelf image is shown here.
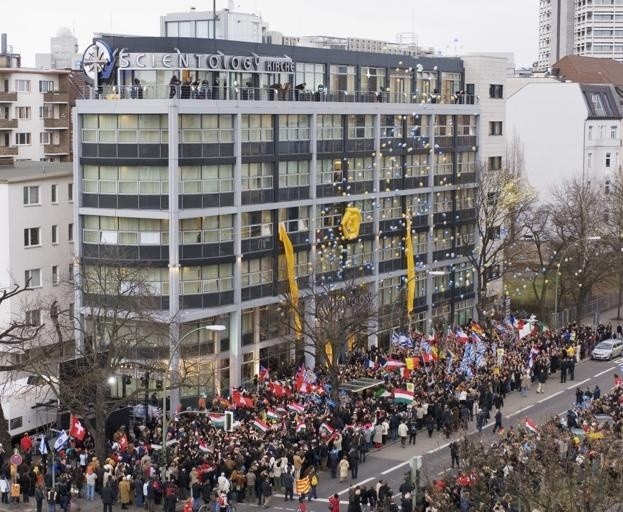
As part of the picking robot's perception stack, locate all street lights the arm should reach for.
[554,235,602,331]
[161,323,227,465]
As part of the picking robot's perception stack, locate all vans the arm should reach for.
[590,338,622,361]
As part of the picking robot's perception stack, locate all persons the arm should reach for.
[131,77,324,102]
[375,85,469,105]
[1,315,623,512]
